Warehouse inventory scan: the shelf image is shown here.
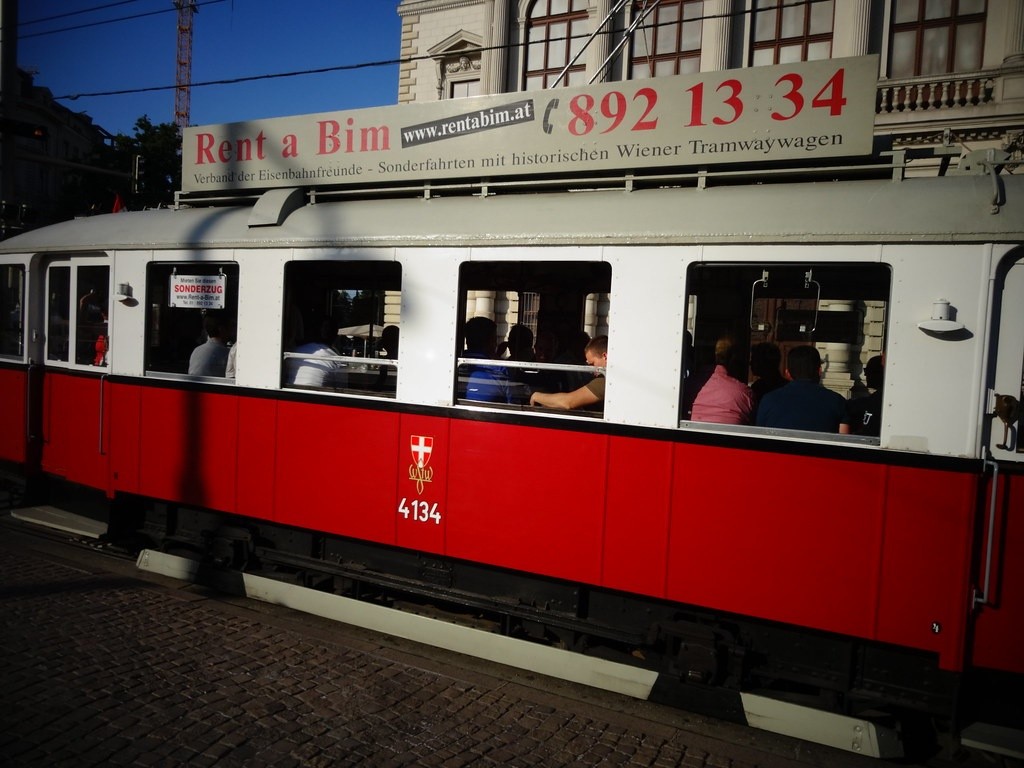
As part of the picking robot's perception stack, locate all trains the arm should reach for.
[0,53,1024,709]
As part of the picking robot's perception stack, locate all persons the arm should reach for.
[679,329,887,438]
[283,314,400,393]
[458,317,608,414]
[47,293,109,363]
[144,308,237,378]
